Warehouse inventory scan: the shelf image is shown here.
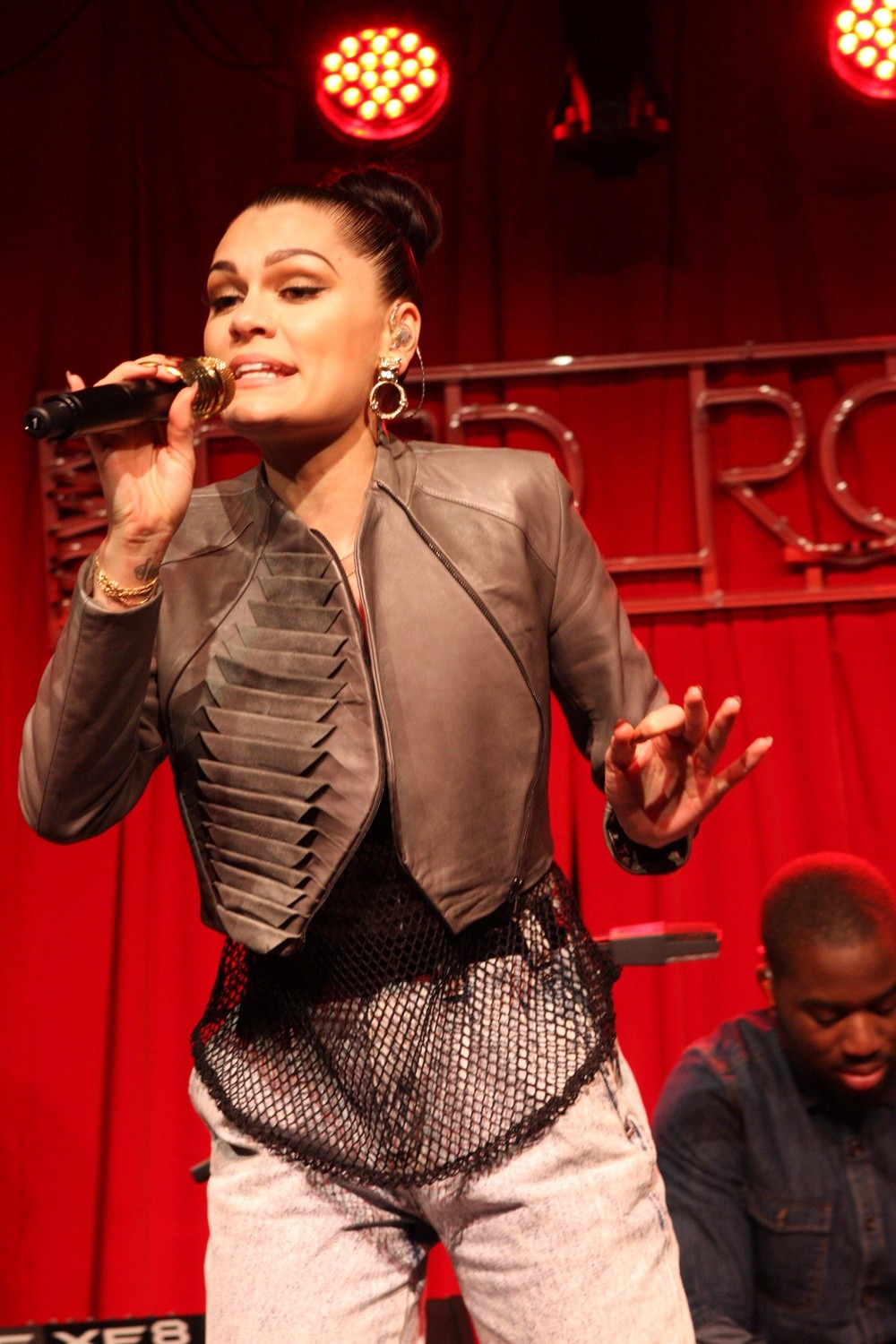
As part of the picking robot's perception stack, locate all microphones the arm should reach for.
[28,352,236,436]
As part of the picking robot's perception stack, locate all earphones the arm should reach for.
[388,303,414,349]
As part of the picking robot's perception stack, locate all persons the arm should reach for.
[19,160,771,1344]
[650,851,896,1343]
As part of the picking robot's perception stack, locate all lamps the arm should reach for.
[549,0,673,179]
[312,20,454,157]
[824,0,896,104]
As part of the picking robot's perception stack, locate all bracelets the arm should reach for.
[87,553,164,612]
